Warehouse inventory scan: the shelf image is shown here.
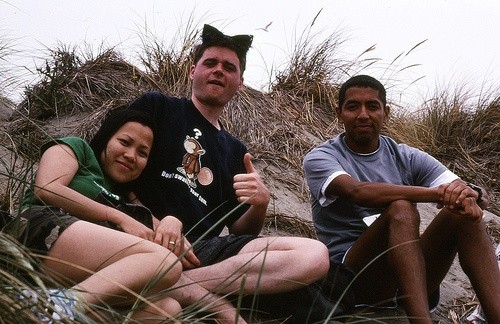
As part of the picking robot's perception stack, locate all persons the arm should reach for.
[12,109,182,324]
[126,24,330,324]
[303,75,499,324]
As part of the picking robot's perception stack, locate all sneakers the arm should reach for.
[28,287,78,324]
[464,302,492,324]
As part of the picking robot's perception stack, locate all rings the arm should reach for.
[169,241,175,246]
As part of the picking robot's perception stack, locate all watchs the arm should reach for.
[465,182,483,205]
